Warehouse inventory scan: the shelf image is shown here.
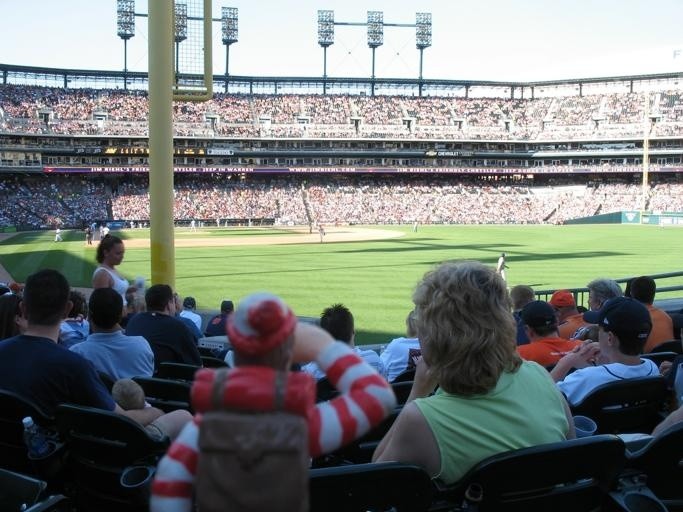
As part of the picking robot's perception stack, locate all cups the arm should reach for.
[135,278,145,293]
[570,416,597,439]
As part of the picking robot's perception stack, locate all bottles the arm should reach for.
[22,417,51,457]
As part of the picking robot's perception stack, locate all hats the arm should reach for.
[583,296,652,339]
[547,290,576,307]
[518,298,555,327]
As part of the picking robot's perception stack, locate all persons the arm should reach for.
[370,258,577,486]
[1,83,683,232]
[511,276,683,408]
[84,225,93,245]
[492,252,510,281]
[103,224,109,237]
[318,223,325,245]
[90,234,138,335]
[54,225,63,242]
[99,223,104,240]
[1,268,397,512]
